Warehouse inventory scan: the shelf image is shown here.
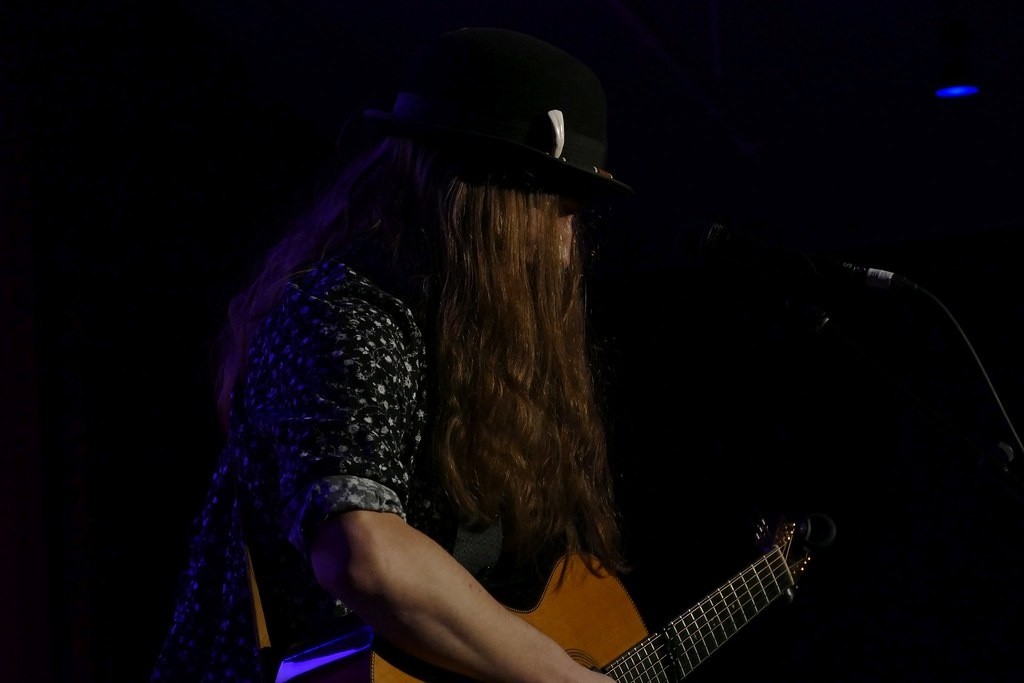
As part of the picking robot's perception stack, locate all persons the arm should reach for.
[155,27,631,683]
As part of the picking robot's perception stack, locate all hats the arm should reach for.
[364,29,636,204]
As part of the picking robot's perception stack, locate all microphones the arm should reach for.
[698,221,919,292]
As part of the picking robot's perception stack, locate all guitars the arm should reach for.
[276,512,839,683]
